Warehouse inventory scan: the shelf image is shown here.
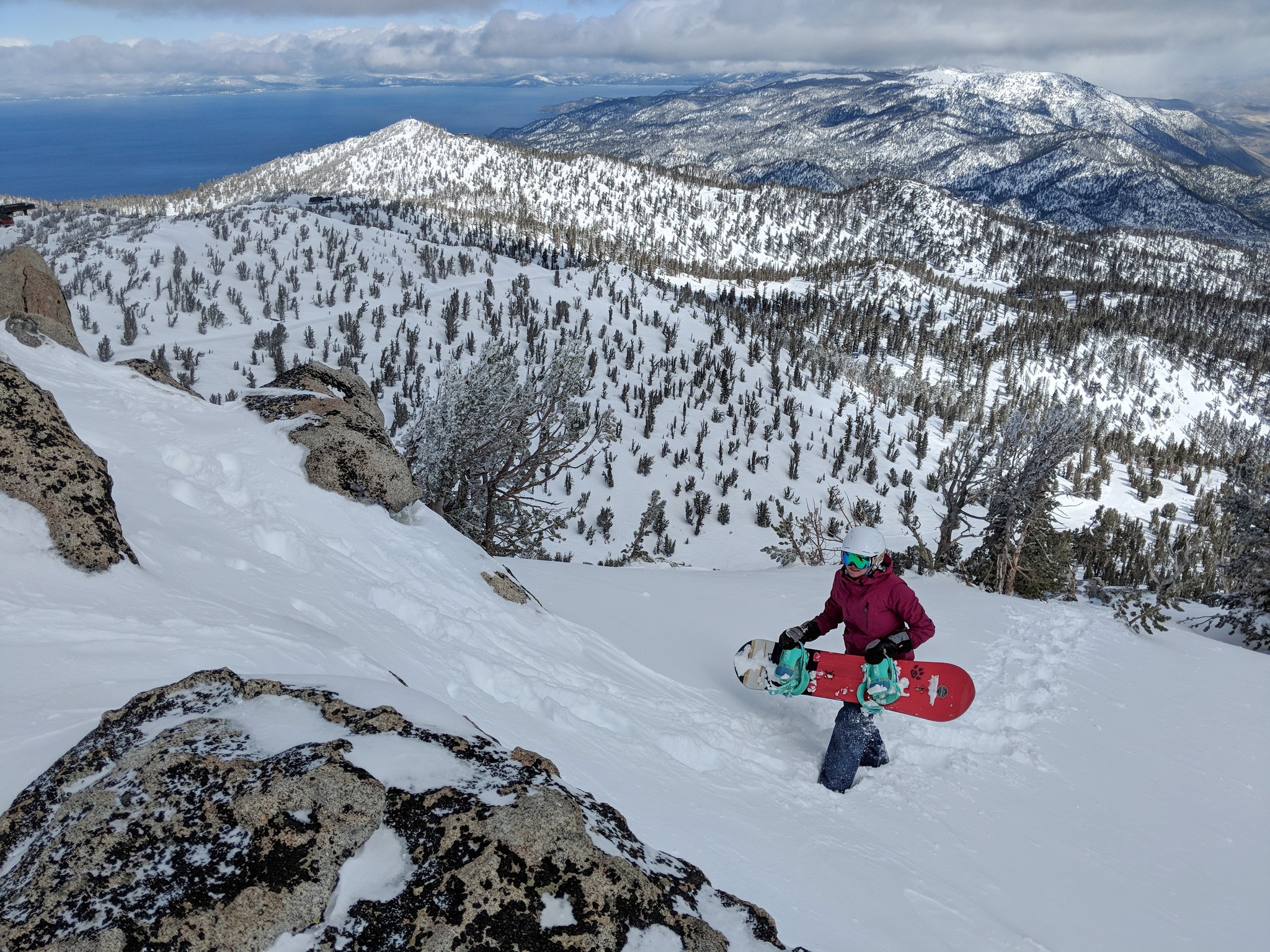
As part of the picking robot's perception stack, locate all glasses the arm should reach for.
[841,552,871,570]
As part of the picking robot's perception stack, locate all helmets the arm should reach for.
[841,526,886,570]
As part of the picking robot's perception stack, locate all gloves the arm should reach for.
[865,631,912,665]
[779,620,821,650]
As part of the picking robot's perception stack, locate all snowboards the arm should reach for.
[734,638,976,722]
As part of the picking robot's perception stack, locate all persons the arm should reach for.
[778,526,935,795]
